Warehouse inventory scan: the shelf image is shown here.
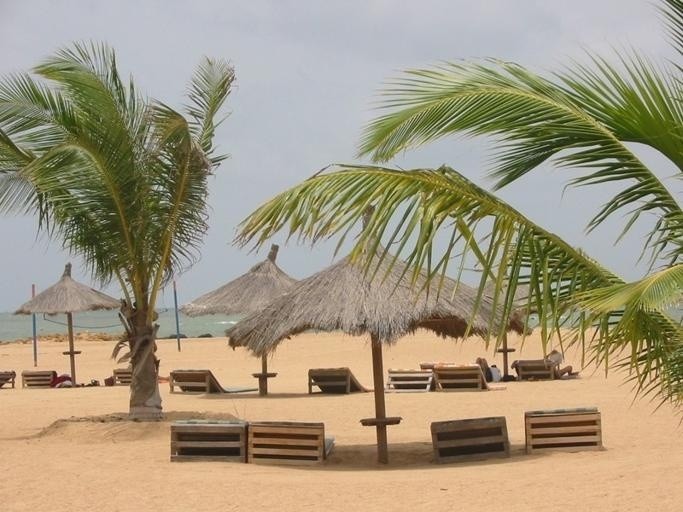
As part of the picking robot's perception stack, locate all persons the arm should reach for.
[544,349,579,379]
[476,357,500,383]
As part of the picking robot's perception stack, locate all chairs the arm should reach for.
[111,366,133,384]
[384,361,491,393]
[0,369,57,386]
[429,405,606,466]
[167,365,259,396]
[515,359,560,381]
[305,365,373,393]
[169,418,334,467]
